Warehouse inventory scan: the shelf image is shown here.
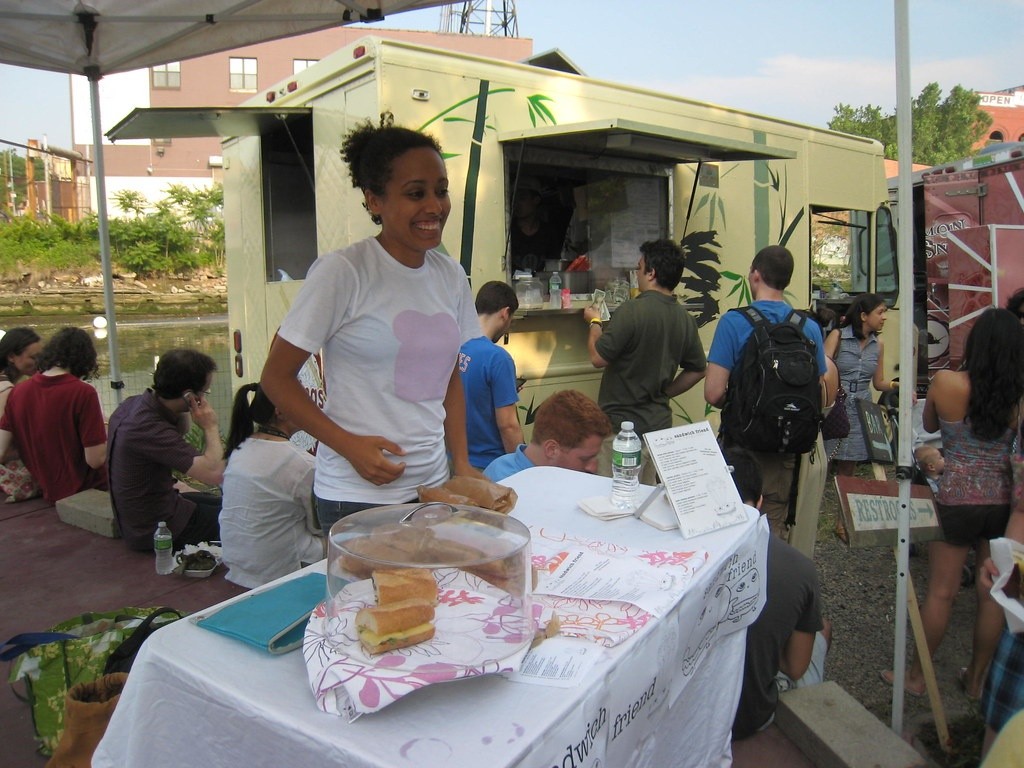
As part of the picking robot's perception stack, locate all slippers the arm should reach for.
[881,670,928,697]
[960,667,981,701]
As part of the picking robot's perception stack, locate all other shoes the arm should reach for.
[835,527,848,544]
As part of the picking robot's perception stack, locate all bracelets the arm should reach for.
[104,422,108,425]
[590,318,602,323]
[890,381,894,390]
[589,322,604,331]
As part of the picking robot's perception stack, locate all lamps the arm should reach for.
[157,146,165,155]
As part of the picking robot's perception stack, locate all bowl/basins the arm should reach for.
[182,554,217,578]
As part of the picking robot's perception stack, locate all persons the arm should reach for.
[0,327,110,507]
[485,389,611,483]
[721,447,832,740]
[704,246,827,541]
[218,381,327,589]
[977,285,1024,768]
[260,122,493,532]
[914,445,945,489]
[823,293,917,544]
[584,240,706,487]
[510,177,565,279]
[0,327,41,419]
[106,347,223,548]
[880,306,1024,701]
[445,280,523,472]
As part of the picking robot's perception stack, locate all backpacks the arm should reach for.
[720,307,823,454]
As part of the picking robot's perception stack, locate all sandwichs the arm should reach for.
[337,523,526,655]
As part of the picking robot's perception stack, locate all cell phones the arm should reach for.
[184,392,201,409]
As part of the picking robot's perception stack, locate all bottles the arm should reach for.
[549,271,571,309]
[603,276,630,308]
[611,421,641,509]
[153,522,172,575]
[812,281,849,300]
[516,277,544,309]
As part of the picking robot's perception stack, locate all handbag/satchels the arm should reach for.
[0,605,193,756]
[822,355,850,440]
[44,672,131,768]
[103,607,185,674]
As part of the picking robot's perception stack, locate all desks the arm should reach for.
[90,464,770,768]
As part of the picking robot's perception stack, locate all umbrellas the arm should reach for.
[0,0,470,408]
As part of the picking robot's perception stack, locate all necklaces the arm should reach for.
[256,426,290,441]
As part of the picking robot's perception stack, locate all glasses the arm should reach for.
[195,388,211,395]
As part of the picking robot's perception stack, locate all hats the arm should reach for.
[510,175,539,190]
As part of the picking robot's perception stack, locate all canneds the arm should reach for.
[561,288,571,309]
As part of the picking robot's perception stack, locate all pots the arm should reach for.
[537,270,593,293]
